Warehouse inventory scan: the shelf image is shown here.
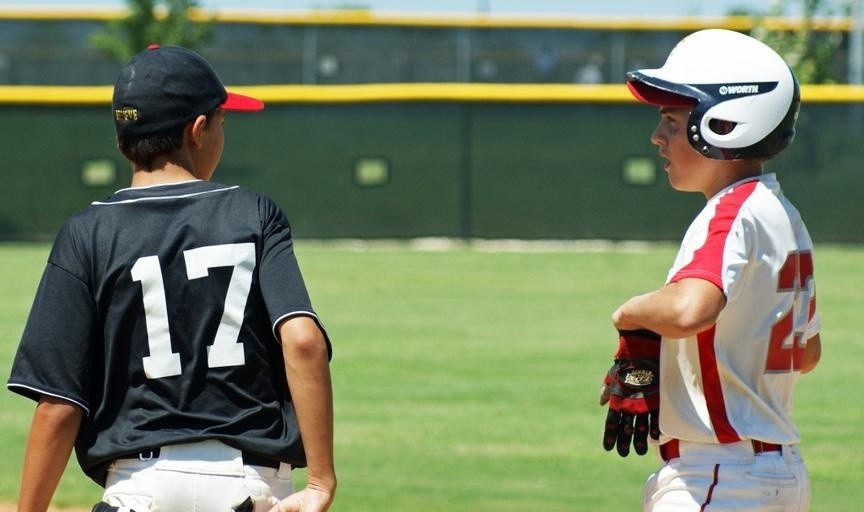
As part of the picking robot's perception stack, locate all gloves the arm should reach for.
[598,329,662,455]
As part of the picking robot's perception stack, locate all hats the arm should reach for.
[111,44,264,134]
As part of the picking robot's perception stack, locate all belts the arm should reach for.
[656,440,782,459]
[116,444,282,470]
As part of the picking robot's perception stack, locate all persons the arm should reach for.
[608,27,828,512]
[3,45,340,512]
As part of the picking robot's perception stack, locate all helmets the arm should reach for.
[625,26,802,163]
